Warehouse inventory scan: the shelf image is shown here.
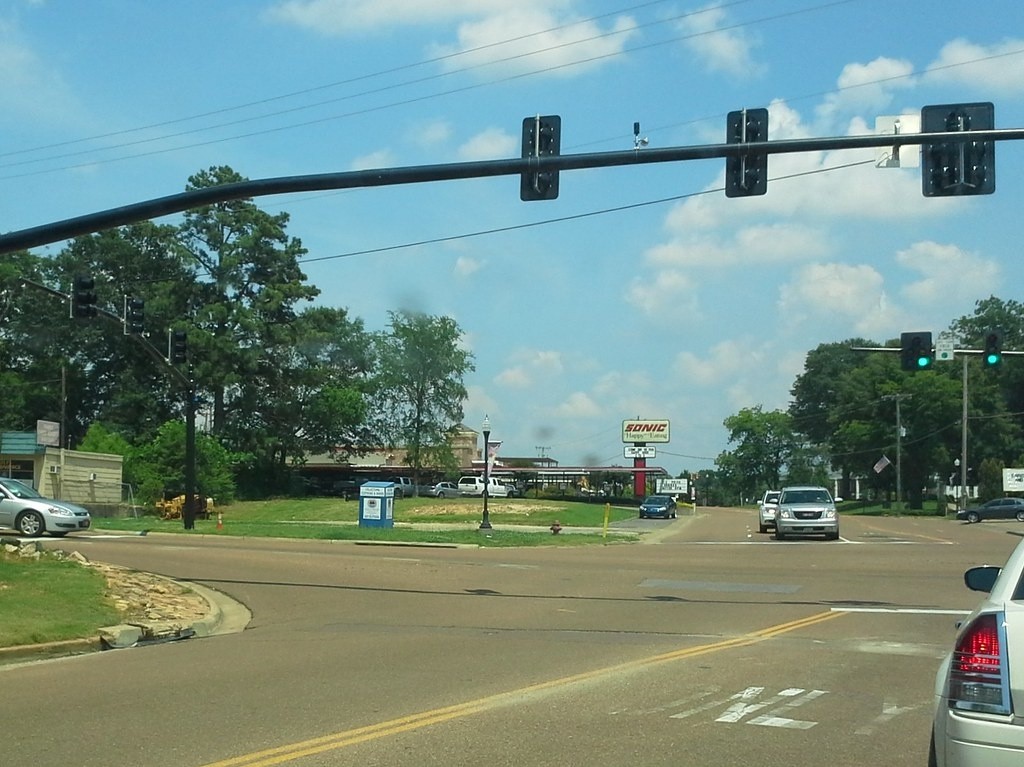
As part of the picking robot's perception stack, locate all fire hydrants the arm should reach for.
[549,520,563,535]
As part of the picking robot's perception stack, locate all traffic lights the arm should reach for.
[124,294,146,334]
[169,327,187,364]
[70,274,98,319]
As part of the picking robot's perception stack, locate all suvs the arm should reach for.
[769,486,844,540]
[458,476,518,499]
[756,490,781,533]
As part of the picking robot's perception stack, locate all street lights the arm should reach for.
[479,412,492,528]
[984,330,1003,366]
[899,331,931,370]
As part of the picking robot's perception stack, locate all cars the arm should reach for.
[422,480,459,499]
[928,537,1023,766]
[386,476,424,499]
[639,495,678,519]
[0,477,92,538]
[955,497,1024,524]
